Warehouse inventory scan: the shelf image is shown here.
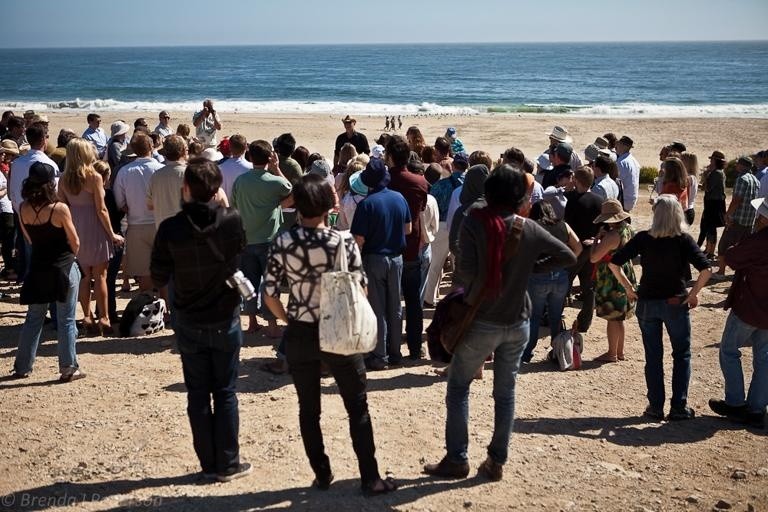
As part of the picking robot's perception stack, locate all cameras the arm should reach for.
[208,105,215,114]
[227,271,255,300]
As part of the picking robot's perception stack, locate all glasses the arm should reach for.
[162,116,170,119]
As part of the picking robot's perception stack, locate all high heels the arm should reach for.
[98,316,113,336]
[82,317,98,335]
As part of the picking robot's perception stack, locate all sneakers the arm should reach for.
[594,353,618,362]
[201,469,218,478]
[644,404,665,419]
[710,271,727,281]
[217,462,254,482]
[750,407,767,427]
[708,397,751,423]
[669,405,696,421]
[619,353,626,361]
[60,368,87,382]
[14,369,30,377]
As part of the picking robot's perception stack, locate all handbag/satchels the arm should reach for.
[181,211,257,303]
[317,235,381,357]
[554,319,580,369]
[426,215,529,369]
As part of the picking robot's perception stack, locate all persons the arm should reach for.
[583,132,616,200]
[333,143,358,200]
[154,110,176,137]
[176,123,224,161]
[384,115,390,130]
[331,115,370,174]
[217,134,253,208]
[697,151,728,255]
[423,164,577,480]
[290,147,333,180]
[145,134,189,232]
[0,111,15,135]
[422,153,469,308]
[90,160,124,323]
[583,198,639,364]
[536,126,581,175]
[523,157,544,209]
[542,167,576,221]
[648,141,698,226]
[708,194,767,425]
[262,173,397,496]
[607,194,712,422]
[58,138,126,336]
[566,166,602,334]
[542,142,575,191]
[232,139,293,337]
[192,99,221,148]
[519,198,583,363]
[496,147,535,219]
[0,116,27,157]
[386,135,429,362]
[759,149,768,205]
[14,161,87,382]
[151,158,252,482]
[397,115,403,130]
[615,136,640,212]
[709,156,760,281]
[536,142,557,177]
[10,122,60,326]
[268,132,303,228]
[406,126,427,160]
[447,151,492,232]
[113,130,166,328]
[0,146,13,280]
[30,114,55,157]
[214,136,235,164]
[435,136,454,173]
[445,127,466,159]
[372,132,391,159]
[337,152,371,201]
[753,150,766,180]
[349,157,411,371]
[133,117,151,135]
[24,110,40,129]
[99,121,130,186]
[49,127,78,173]
[390,116,395,130]
[449,164,490,254]
[82,113,110,160]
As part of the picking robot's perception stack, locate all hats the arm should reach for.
[544,125,573,143]
[598,148,617,164]
[349,170,369,195]
[593,199,631,224]
[421,162,451,184]
[617,136,634,147]
[593,136,610,149]
[111,121,130,136]
[32,114,48,123]
[553,141,573,156]
[361,158,391,189]
[750,196,768,218]
[342,115,356,124]
[1,139,20,155]
[19,142,31,153]
[707,149,726,163]
[202,148,224,162]
[23,110,35,118]
[120,140,137,158]
[584,144,600,161]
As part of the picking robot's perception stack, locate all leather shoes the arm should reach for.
[424,457,470,478]
[477,457,502,482]
[411,347,426,358]
[435,364,451,377]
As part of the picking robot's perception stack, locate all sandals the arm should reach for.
[315,472,334,490]
[363,478,398,494]
